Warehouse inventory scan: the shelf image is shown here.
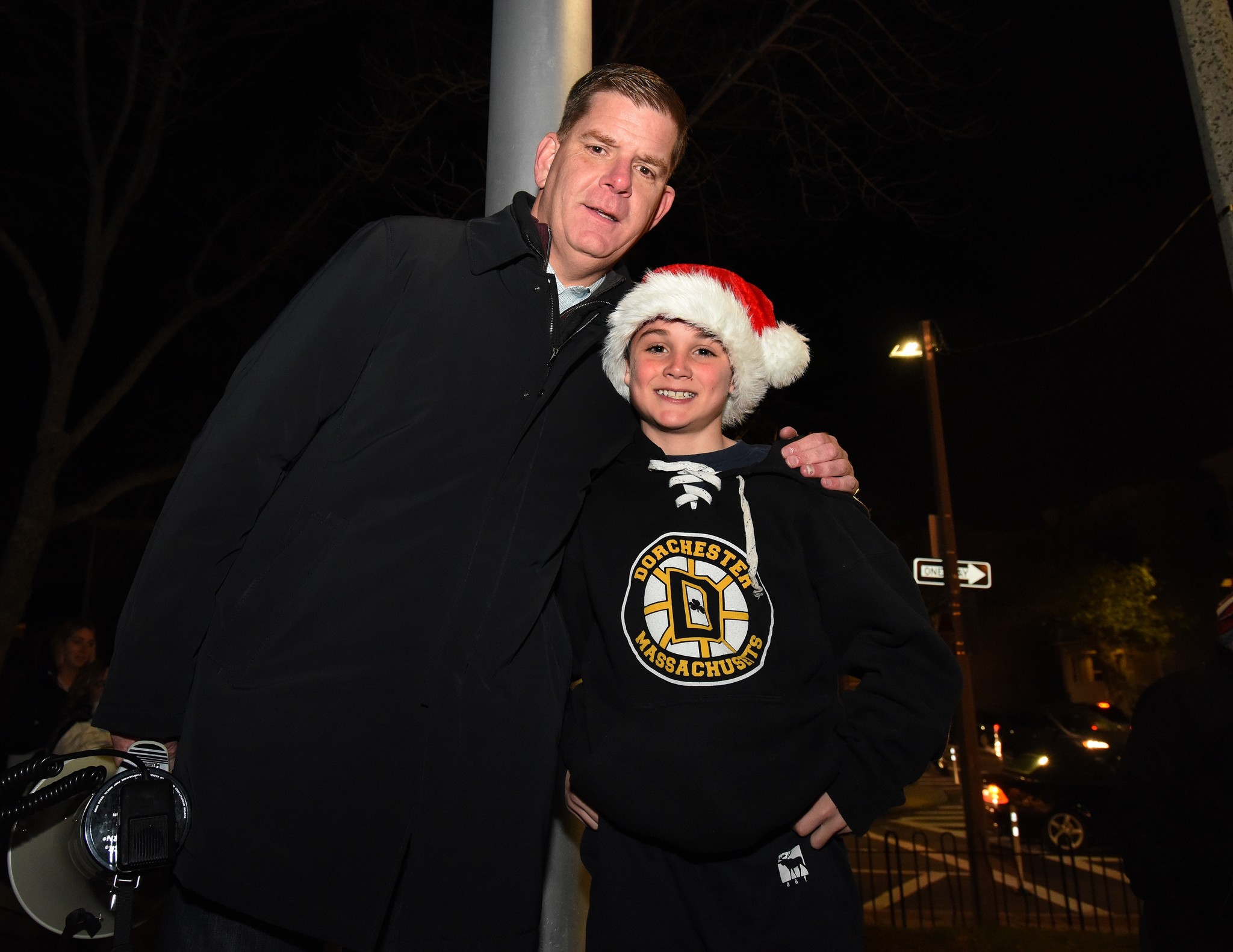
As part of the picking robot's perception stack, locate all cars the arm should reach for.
[933,703,1141,857]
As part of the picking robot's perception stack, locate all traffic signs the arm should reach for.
[913,555,993,589]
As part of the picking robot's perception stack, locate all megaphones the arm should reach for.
[8,741,191,939]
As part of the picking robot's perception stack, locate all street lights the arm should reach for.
[886,318,1000,928]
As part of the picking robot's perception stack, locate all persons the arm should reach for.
[0,618,96,773]
[1109,591,1233,952]
[90,63,858,952]
[553,263,962,952]
[44,659,109,753]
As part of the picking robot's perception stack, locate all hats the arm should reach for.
[601,263,811,430]
